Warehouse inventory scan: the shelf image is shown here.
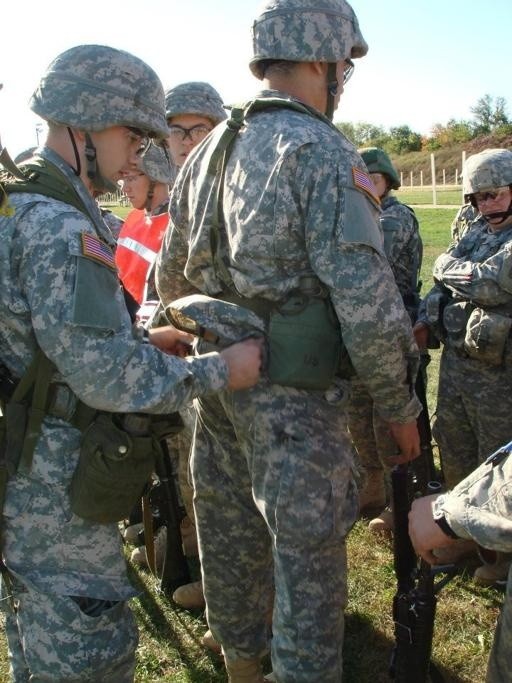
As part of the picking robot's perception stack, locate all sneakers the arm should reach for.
[122,512,217,650]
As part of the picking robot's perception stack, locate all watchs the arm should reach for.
[432,496,456,541]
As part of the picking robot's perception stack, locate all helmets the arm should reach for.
[462,149,512,204]
[248,0,369,81]
[357,147,401,190]
[28,44,170,140]
[134,136,176,183]
[165,82,228,126]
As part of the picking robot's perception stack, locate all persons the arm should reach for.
[0,43,266,683]
[353,147,433,532]
[450,203,478,243]
[412,148,512,489]
[408,438,512,683]
[113,137,174,327]
[97,207,124,245]
[162,79,228,170]
[156,0,421,682]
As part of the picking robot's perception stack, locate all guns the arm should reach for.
[137,439,190,594]
[387,352,438,681]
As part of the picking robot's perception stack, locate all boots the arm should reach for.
[357,467,395,533]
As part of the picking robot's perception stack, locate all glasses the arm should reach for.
[168,125,210,142]
[343,57,354,86]
[465,186,511,204]
